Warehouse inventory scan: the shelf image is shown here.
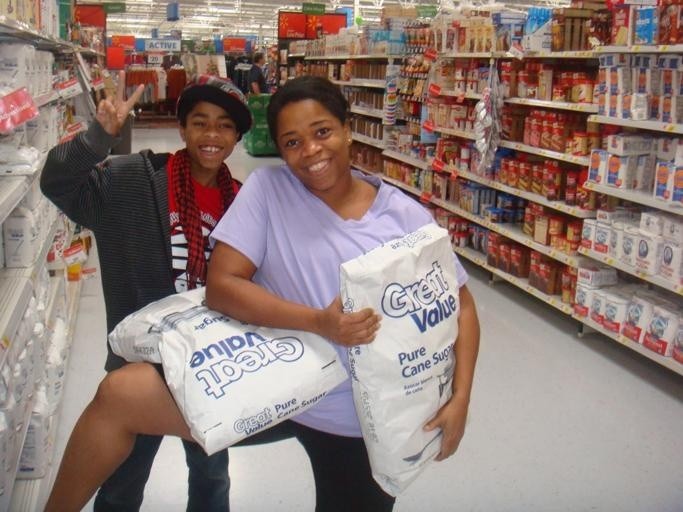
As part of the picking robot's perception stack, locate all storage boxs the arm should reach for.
[242,94,279,158]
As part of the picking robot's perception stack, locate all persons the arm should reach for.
[246,53,269,95]
[42,73,480,512]
[38,69,255,512]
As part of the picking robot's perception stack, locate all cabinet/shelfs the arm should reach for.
[306,44,594,320]
[0,15,106,512]
[576,44,683,376]
[276,45,305,87]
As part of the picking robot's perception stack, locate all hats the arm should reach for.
[176,73,252,141]
